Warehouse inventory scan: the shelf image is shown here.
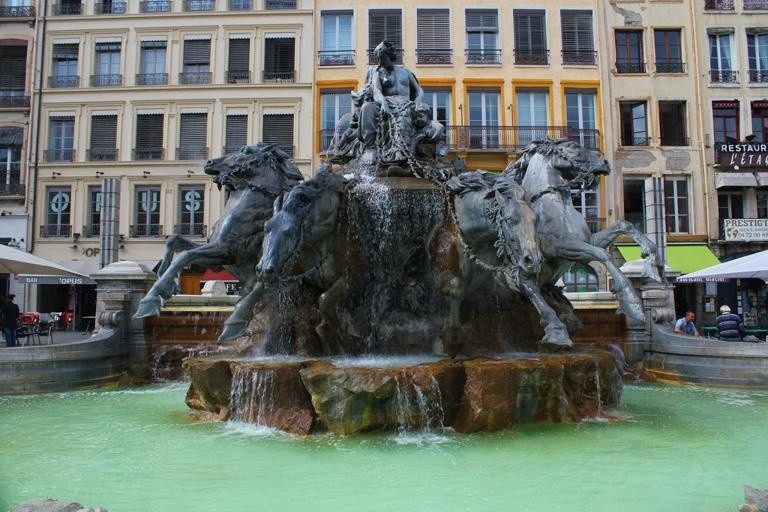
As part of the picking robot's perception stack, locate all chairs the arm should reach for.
[11,307,75,345]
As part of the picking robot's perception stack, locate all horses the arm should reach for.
[133,142,307,321]
[253,163,348,344]
[444,165,576,350]
[499,136,668,324]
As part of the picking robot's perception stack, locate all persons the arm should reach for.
[349,37,427,164]
[0,294,19,347]
[715,304,744,342]
[674,311,700,339]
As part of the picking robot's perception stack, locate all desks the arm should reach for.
[81,314,96,336]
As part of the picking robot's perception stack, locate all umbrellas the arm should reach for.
[0,244,82,276]
[675,249,768,285]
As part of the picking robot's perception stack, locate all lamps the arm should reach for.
[51,171,61,179]
[142,170,151,180]
[1,207,12,218]
[186,168,193,177]
[96,171,105,179]
